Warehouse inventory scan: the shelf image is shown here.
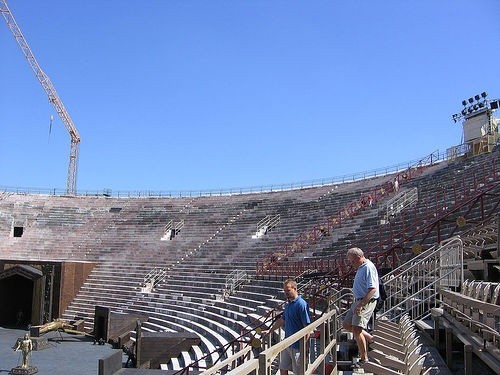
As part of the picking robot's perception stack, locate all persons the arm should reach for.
[393,177,399,192]
[15,334,32,368]
[341,248,381,370]
[262,278,312,375]
[359,193,373,207]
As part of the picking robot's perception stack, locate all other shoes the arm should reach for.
[368,338,381,352]
[350,358,370,369]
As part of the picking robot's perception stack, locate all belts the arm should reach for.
[354,297,364,301]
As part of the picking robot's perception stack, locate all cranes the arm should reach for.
[0,0,80,195]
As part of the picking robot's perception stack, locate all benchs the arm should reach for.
[0,149,500,375]
